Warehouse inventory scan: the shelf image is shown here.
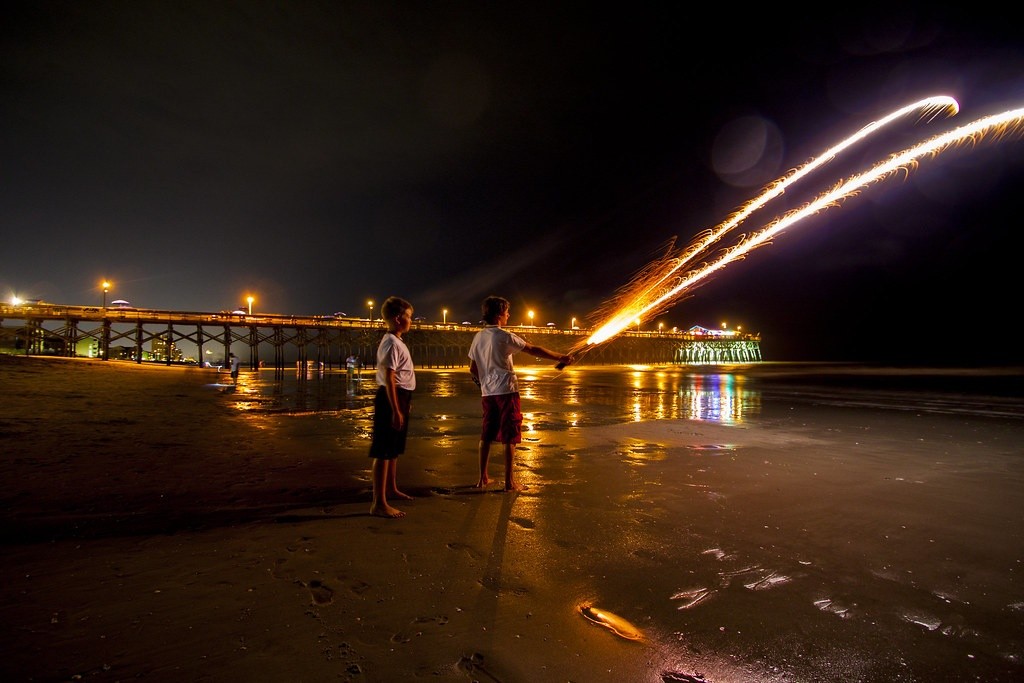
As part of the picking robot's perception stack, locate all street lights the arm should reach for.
[571,317,576,329]
[367,301,373,321]
[528,311,534,326]
[102,282,109,309]
[247,296,255,315]
[443,309,448,324]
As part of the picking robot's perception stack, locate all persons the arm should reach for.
[229,353,240,387]
[468,297,575,492]
[368,296,416,518]
[346,354,363,382]
